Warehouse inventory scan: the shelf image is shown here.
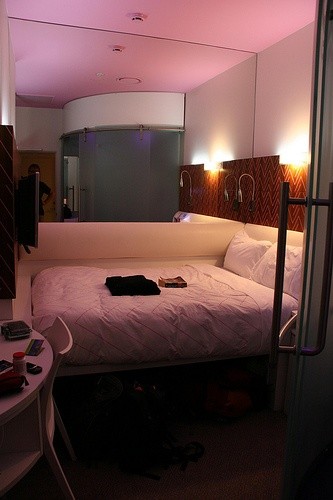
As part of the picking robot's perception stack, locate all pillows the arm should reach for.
[224,230,273,278]
[250,242,303,299]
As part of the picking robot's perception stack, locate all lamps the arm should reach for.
[237,174,256,219]
[223,174,238,204]
[180,169,193,206]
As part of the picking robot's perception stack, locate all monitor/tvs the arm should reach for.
[18,171,39,249]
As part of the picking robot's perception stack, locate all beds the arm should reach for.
[31,223,303,410]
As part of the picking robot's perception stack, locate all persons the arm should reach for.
[19,164,56,222]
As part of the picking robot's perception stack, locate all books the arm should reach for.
[158,277,187,288]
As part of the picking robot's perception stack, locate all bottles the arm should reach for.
[12,352,27,376]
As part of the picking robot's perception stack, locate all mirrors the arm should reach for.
[7,16,259,224]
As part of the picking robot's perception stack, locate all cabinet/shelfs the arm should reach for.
[0,327,54,498]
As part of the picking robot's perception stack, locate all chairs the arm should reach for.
[37,315,76,500]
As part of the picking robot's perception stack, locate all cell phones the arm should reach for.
[26,361,41,374]
